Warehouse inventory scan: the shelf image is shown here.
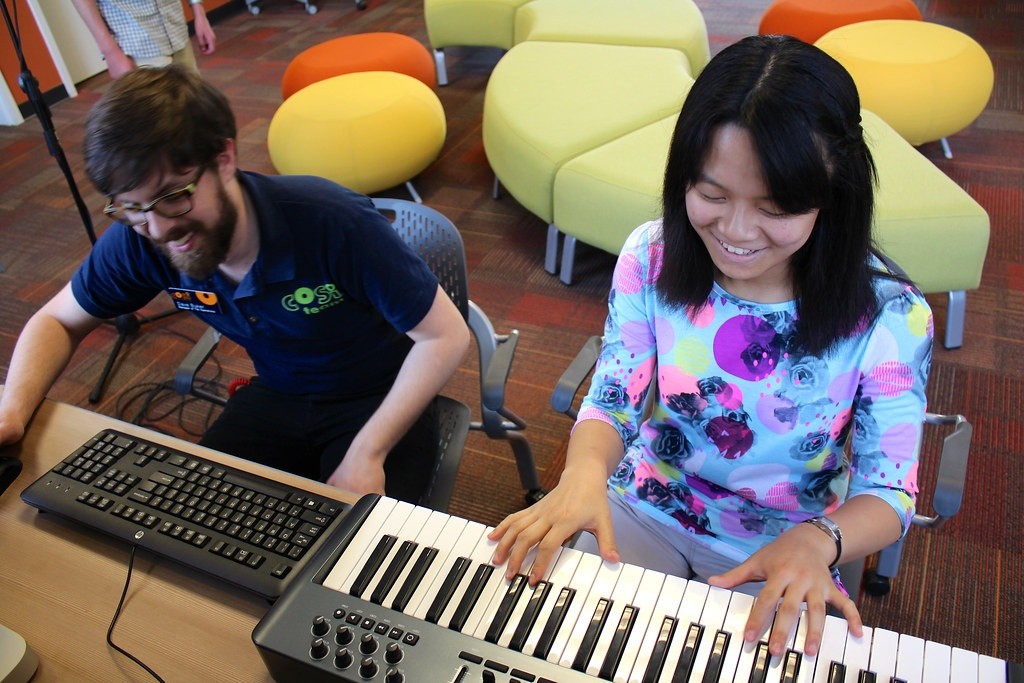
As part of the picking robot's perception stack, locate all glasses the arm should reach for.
[102,160,209,228]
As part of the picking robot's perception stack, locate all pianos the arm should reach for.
[247,488,1024,683]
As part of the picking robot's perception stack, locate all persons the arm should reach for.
[487,36,934,656]
[71,0,217,80]
[0,65,472,495]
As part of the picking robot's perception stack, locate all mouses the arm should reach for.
[0,455,22,496]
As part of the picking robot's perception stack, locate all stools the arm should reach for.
[281,31,435,104]
[758,0,922,44]
[423,0,531,86]
[482,41,697,274]
[858,107,990,351]
[267,71,448,204]
[552,111,681,286]
[514,0,714,79]
[811,20,995,159]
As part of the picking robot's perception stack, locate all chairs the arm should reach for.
[175,197,546,516]
[551,247,974,596]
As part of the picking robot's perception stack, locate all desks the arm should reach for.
[0,398,360,683]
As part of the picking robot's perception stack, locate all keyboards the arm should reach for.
[19,428,354,603]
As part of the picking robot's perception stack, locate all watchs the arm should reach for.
[802,516,843,567]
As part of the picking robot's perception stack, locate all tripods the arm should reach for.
[0,0,178,406]
